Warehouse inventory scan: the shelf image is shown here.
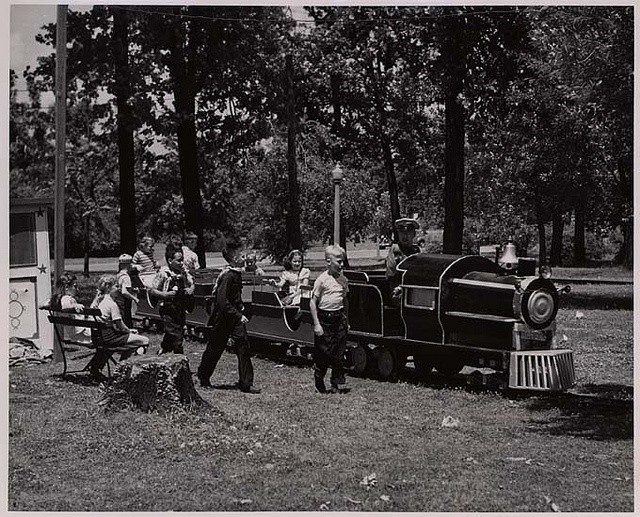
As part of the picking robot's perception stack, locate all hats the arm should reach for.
[119,254,132,263]
[394,218,419,230]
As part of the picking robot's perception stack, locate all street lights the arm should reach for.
[332,162,344,246]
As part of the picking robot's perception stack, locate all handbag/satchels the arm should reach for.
[281,292,294,306]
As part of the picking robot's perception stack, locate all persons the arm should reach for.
[163,234,199,271]
[111,253,140,328]
[385,218,420,307]
[309,244,349,394]
[44,273,112,381]
[267,249,311,307]
[150,245,195,356]
[90,274,149,359]
[131,236,161,308]
[198,242,262,393]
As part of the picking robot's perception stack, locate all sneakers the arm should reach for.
[331,384,338,392]
[198,372,212,389]
[315,381,327,394]
[239,385,261,393]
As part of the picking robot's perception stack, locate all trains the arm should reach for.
[134,234,577,394]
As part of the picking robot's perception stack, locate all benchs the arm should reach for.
[40,304,148,380]
[253,291,302,327]
[343,266,402,336]
[190,269,217,293]
